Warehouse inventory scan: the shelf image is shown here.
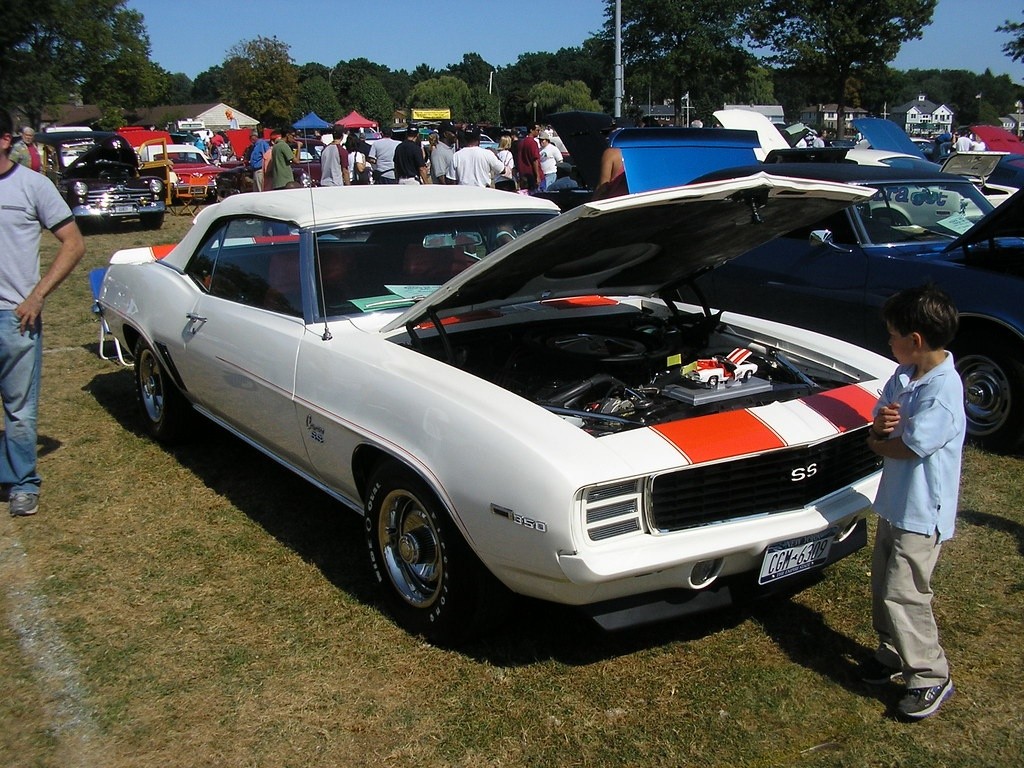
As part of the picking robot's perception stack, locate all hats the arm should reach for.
[464,123,483,137]
[537,133,549,140]
[437,121,461,133]
[408,125,419,132]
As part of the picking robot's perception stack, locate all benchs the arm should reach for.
[200,242,390,300]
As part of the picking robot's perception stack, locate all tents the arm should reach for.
[293,112,335,129]
[335,111,379,133]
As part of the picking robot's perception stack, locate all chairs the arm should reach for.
[404,242,469,285]
[174,174,210,217]
[262,249,314,316]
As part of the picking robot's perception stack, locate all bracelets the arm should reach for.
[869,427,885,441]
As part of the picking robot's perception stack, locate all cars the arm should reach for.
[8,130,169,230]
[590,118,1024,449]
[116,106,1024,221]
[95,172,921,647]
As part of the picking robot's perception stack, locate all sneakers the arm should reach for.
[10,491,40,516]
[860,663,903,685]
[898,675,953,717]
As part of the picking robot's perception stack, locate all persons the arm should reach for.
[0,109,86,516]
[9,122,988,201]
[865,288,967,717]
[263,182,301,237]
[494,220,519,249]
